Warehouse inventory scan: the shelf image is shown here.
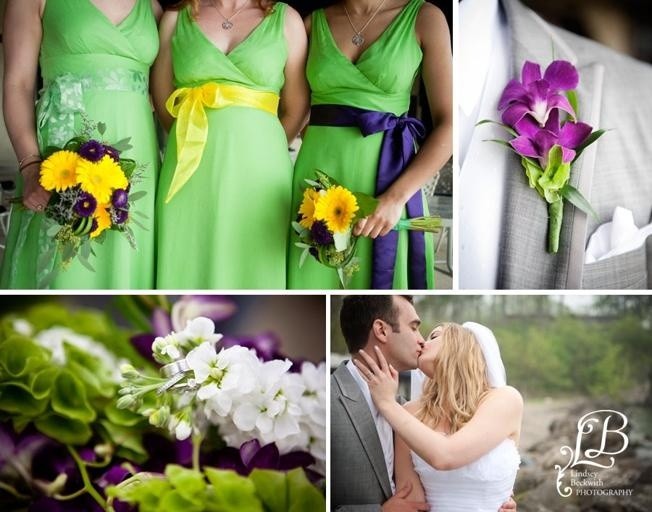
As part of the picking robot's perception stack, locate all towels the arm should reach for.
[582,204,652,265]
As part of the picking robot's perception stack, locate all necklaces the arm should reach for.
[339,0,387,45]
[210,0,251,29]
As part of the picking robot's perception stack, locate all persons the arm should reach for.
[0,0,164,290]
[327,295,519,512]
[352,320,525,512]
[290,0,451,290]
[145,0,311,290]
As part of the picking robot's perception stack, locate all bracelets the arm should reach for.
[16,153,41,172]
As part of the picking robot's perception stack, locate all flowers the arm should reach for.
[471,41,615,256]
[292,166,444,289]
[0,297,325,511]
[6,132,148,274]
